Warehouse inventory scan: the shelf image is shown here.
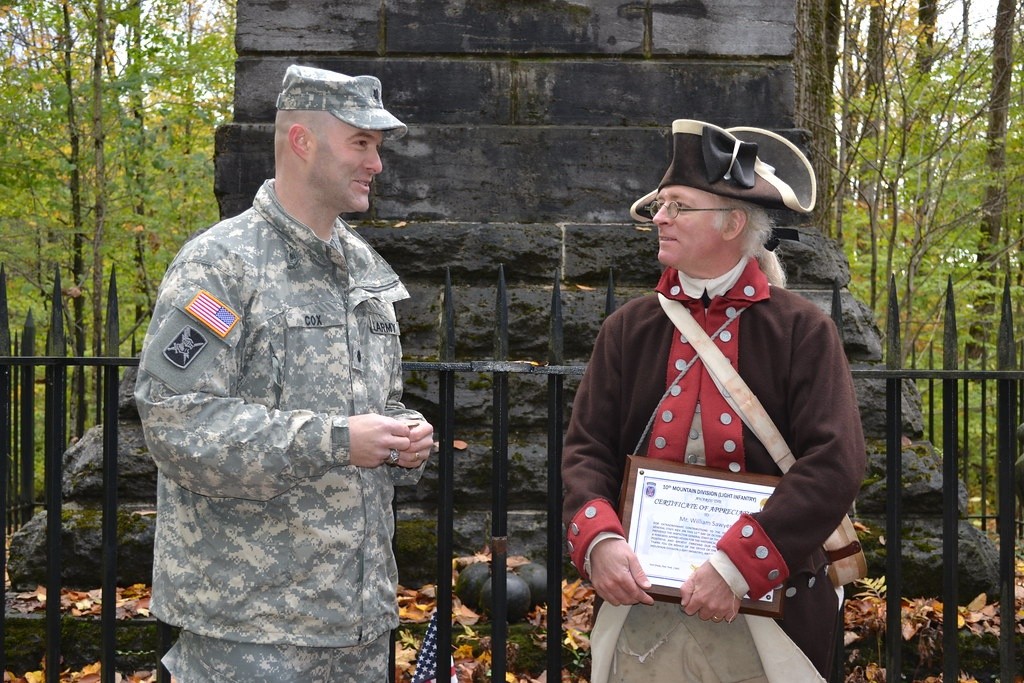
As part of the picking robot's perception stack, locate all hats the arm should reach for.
[629,118,816,223]
[275,62,409,141]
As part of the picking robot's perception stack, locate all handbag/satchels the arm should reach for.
[823,518,868,589]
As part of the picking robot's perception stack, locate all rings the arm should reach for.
[712,615,723,621]
[414,452,420,462]
[387,450,399,466]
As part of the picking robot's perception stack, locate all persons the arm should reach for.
[133,65,435,683]
[561,120,864,683]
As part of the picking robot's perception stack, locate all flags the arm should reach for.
[410,606,457,683]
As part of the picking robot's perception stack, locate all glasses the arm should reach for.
[649,200,741,220]
